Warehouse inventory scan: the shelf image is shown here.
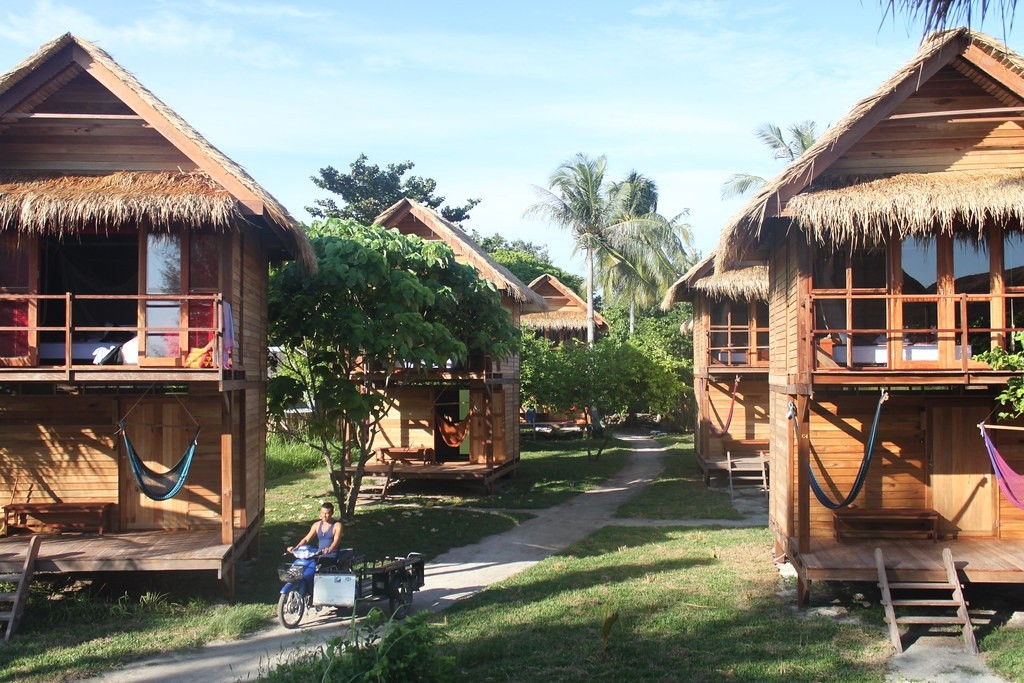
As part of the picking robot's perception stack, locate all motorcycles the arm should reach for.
[278,545,425,631]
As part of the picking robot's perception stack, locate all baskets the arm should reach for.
[277,563,304,582]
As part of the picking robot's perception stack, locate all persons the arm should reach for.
[287,503,344,612]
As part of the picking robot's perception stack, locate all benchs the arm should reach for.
[833,346,972,366]
[2,502,114,536]
[833,508,939,543]
[373,447,433,464]
[719,352,747,362]
[520,412,550,423]
[723,440,770,453]
